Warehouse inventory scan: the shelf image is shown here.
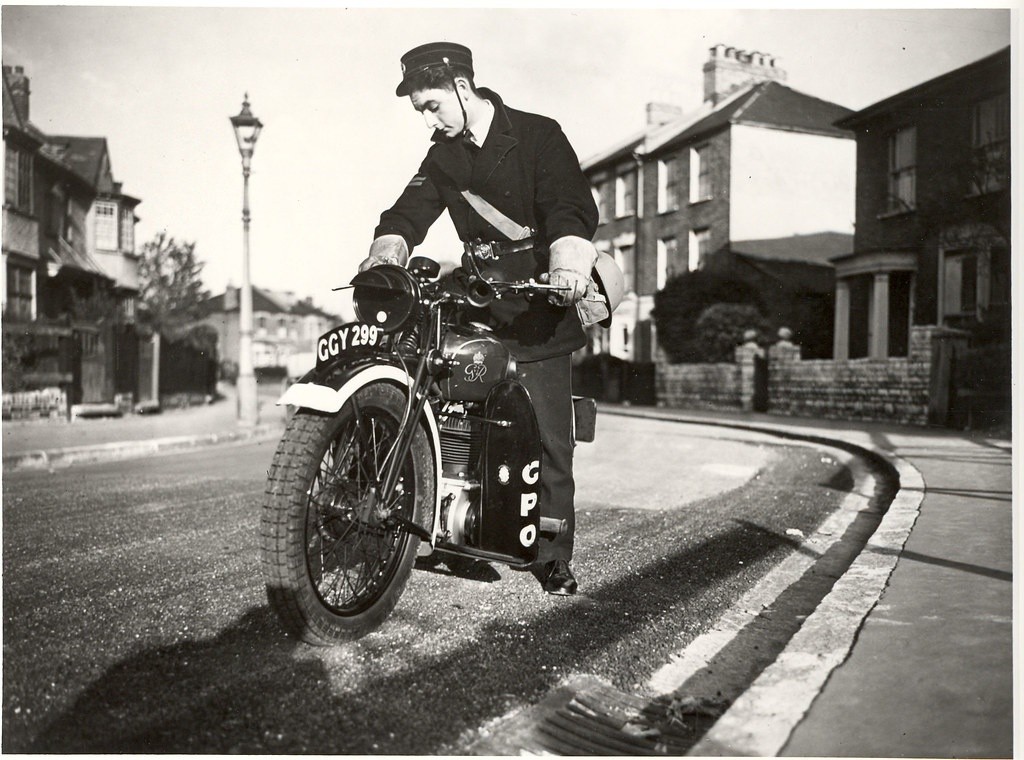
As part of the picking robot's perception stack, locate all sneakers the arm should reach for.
[542,559,578,595]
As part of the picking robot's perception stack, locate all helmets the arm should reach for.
[591,252,624,328]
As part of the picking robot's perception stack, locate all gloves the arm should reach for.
[358,235,409,274]
[548,229,599,307]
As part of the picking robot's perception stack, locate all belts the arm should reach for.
[464,234,536,260]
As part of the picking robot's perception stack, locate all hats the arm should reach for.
[396,42,474,97]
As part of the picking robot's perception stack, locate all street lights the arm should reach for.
[228,90,265,424]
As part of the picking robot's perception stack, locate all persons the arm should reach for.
[358,43,599,597]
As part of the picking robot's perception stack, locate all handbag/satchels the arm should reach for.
[575,276,609,327]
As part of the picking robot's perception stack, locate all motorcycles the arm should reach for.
[258,255,600,642]
